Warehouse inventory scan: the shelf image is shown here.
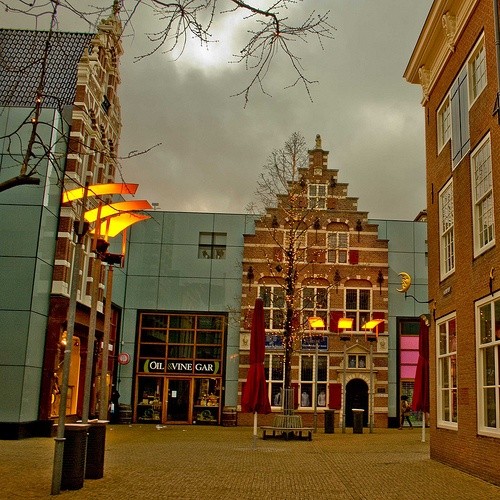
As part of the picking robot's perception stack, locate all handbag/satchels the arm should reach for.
[401,400,410,412]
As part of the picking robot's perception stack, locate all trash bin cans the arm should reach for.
[77,419,109,480]
[56,424,88,490]
[324,409,335,434]
[353,408,364,434]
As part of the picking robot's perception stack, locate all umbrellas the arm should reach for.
[413,316,430,443]
[240,299,272,436]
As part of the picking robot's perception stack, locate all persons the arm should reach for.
[302,391,309,406]
[318,392,325,406]
[273,392,282,406]
[401,395,413,429]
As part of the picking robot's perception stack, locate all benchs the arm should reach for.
[260,425,315,442]
